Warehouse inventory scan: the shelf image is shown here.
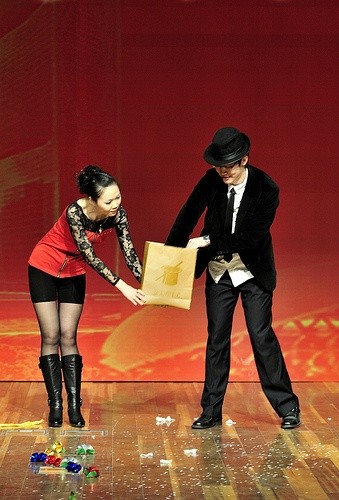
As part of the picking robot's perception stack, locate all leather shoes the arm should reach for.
[191,416,223,428]
[281,407,301,428]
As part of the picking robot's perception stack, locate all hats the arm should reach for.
[203,126,251,167]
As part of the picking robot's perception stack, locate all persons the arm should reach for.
[164,127,303,428]
[28,163,146,427]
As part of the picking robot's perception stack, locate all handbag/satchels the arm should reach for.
[138,241,198,311]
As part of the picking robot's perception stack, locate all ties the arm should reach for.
[222,188,237,264]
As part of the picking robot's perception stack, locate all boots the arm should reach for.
[60,354,86,427]
[39,354,65,427]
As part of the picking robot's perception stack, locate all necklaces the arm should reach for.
[93,217,109,233]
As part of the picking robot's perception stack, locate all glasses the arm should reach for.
[214,163,239,169]
